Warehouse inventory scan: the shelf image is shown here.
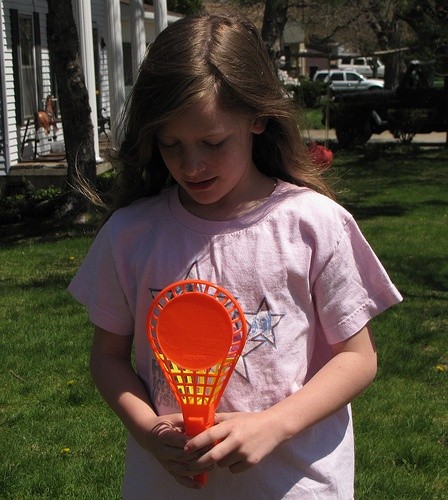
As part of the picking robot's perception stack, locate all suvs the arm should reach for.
[321,59,448,145]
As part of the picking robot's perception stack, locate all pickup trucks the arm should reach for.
[313,69,384,96]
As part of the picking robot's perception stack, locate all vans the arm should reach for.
[336,56,385,78]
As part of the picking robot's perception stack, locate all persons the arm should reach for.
[63,14,403,500]
